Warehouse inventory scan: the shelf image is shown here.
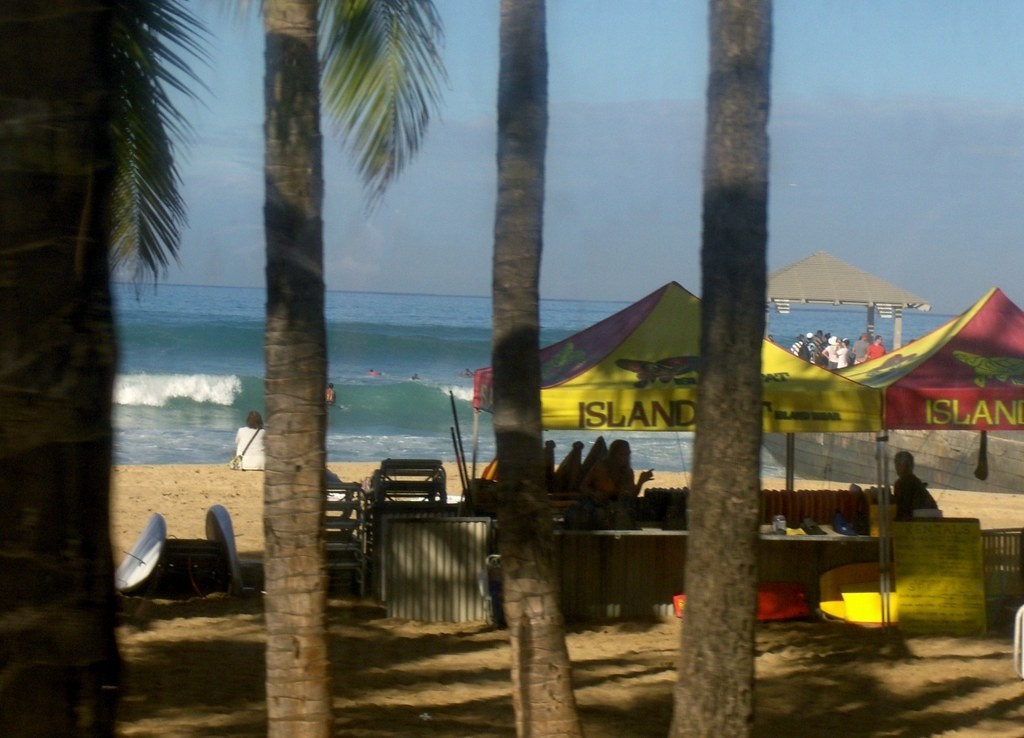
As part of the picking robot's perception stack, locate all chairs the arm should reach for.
[324,457,449,598]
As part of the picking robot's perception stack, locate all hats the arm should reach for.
[828,336,841,345]
[807,332,814,339]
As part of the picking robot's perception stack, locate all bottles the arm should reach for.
[772,514,786,535]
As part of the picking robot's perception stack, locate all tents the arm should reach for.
[829,286,1024,637]
[765,251,932,353]
[474,281,884,641]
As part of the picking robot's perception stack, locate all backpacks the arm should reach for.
[798,344,810,362]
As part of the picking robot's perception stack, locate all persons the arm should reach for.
[235,411,265,470]
[325,383,335,401]
[889,452,944,520]
[791,330,886,369]
[369,368,471,379]
[582,440,654,530]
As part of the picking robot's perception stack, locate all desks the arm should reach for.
[552,518,887,623]
